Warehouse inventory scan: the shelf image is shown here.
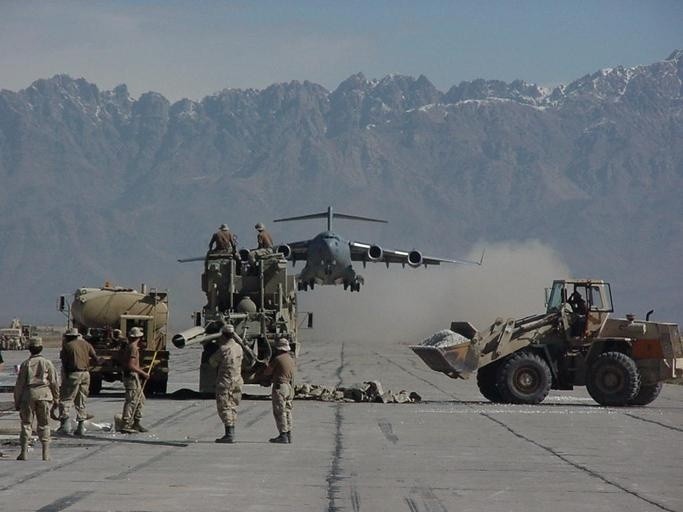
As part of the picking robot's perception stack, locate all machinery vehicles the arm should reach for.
[401,277,681,410]
[171,252,316,398]
[0,318,44,351]
[56,280,173,399]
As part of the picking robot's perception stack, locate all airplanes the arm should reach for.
[174,207,487,294]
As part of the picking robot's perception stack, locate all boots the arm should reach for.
[57,419,70,433]
[74,421,84,434]
[121,419,148,432]
[43,442,49,460]
[269,431,292,443]
[215,426,235,443]
[17,445,27,460]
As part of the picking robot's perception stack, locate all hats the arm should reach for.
[223,324,234,333]
[128,327,144,338]
[65,328,80,336]
[274,338,291,352]
[255,223,264,230]
[218,224,229,231]
[29,336,42,347]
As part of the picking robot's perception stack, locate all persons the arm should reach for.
[11,336,60,462]
[568,290,585,314]
[206,222,234,254]
[56,326,99,440]
[22,328,28,336]
[246,221,273,275]
[1,333,26,351]
[205,323,245,445]
[118,326,151,435]
[260,336,296,445]
